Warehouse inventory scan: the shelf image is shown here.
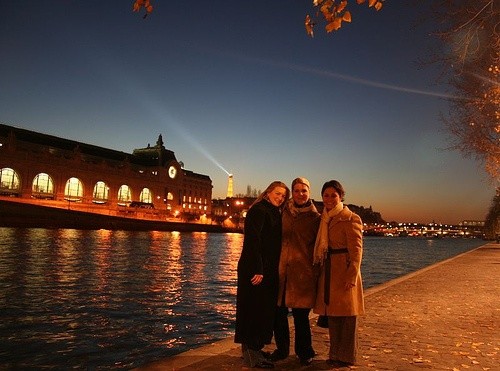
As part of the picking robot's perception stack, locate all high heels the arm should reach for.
[300,357,313,366]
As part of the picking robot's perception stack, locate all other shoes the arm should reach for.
[271,349,289,361]
[254,359,274,368]
[260,351,271,359]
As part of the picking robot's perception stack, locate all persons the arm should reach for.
[268,177,321,366]
[313,180,364,367]
[234,181,290,368]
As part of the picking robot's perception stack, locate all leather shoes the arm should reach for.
[326,359,352,367]
